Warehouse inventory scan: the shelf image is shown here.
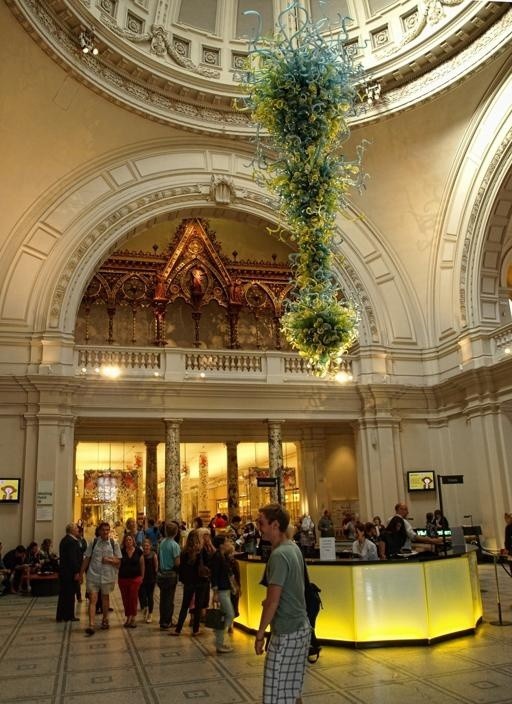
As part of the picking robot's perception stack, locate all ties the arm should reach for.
[0,478,21,503]
[407,471,435,491]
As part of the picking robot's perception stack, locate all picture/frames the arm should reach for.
[22,570,59,597]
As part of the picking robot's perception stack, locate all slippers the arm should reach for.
[304,581,322,657]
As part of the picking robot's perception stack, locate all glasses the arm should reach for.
[78,32,98,57]
[366,83,381,104]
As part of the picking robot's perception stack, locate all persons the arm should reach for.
[2,538,59,594]
[318,502,451,564]
[504,512,512,557]
[254,503,323,704]
[56,512,262,652]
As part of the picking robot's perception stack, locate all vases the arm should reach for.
[232,0,380,376]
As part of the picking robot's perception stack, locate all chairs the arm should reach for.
[124,622,136,628]
[216,645,233,653]
[85,626,95,635]
[102,620,109,629]
[159,624,205,636]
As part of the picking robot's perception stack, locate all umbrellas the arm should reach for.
[70,617,80,622]
[146,614,152,623]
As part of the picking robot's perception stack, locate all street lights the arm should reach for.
[205,608,227,629]
[157,570,176,583]
[198,566,211,578]
